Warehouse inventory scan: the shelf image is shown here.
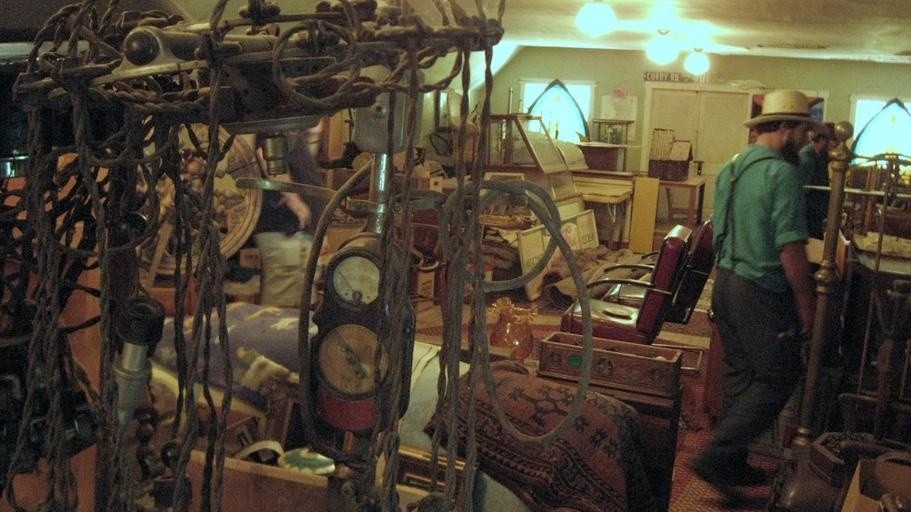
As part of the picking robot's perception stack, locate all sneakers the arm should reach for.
[685,452,782,508]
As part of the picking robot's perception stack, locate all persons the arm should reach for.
[686,86,815,488]
[244,133,327,233]
[801,118,839,230]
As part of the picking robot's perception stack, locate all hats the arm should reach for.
[742,88,819,129]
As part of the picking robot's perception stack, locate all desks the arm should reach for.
[570,169,706,240]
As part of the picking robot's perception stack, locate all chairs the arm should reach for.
[560,218,713,374]
